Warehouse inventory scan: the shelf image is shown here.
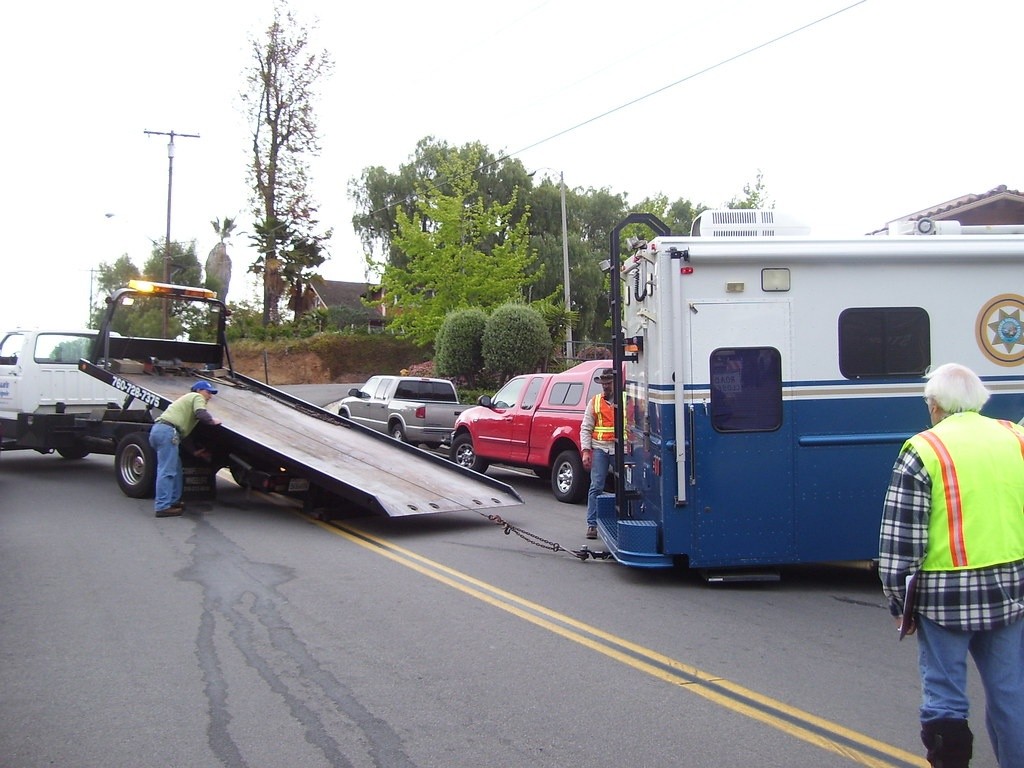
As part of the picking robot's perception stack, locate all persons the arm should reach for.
[877,363,1024,768]
[580,368,627,539]
[149,380,222,517]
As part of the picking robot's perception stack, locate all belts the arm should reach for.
[157,420,179,432]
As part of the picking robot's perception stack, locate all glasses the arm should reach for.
[922,396,928,404]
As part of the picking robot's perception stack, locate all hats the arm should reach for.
[594,368,613,384]
[191,380,218,395]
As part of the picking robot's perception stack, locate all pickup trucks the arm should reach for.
[338,375,477,449]
[449,359,627,503]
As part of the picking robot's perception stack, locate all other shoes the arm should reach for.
[173,500,185,509]
[156,506,183,517]
[586,527,598,539]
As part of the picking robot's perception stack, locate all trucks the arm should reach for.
[0,280,521,517]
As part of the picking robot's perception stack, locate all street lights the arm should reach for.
[106,213,170,339]
